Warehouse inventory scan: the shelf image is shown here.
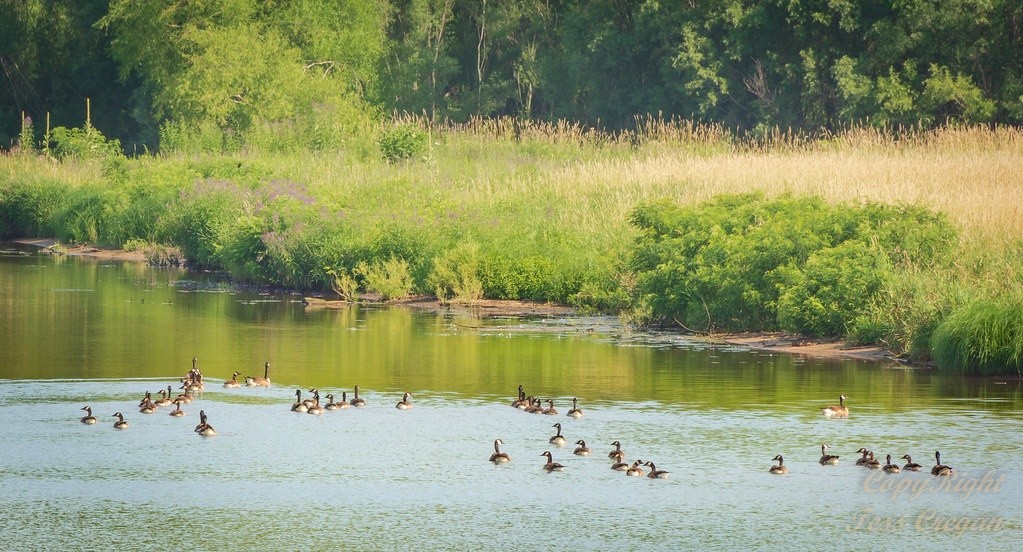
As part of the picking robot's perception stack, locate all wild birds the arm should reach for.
[770,455,788,474]
[549,422,568,448]
[112,412,128,428]
[818,442,840,466]
[574,440,590,456]
[854,447,952,478]
[80,406,96,424]
[396,392,414,410]
[488,439,510,465]
[194,410,216,437]
[608,441,673,480]
[819,393,850,418]
[511,384,558,415]
[541,451,567,473]
[567,397,583,418]
[290,385,367,414]
[137,357,205,417]
[223,361,271,389]
